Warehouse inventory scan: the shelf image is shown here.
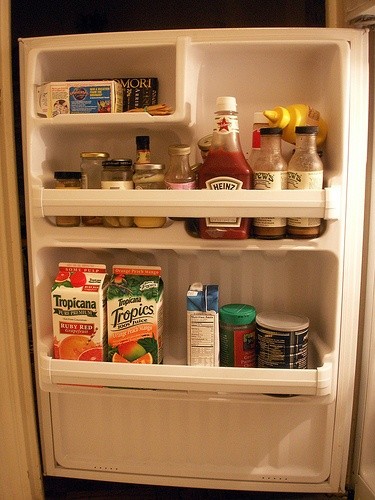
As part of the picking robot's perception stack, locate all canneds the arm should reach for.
[218,304,311,398]
[53,151,167,228]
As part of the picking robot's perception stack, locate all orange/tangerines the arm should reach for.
[55,272,71,282]
[111,353,153,364]
[54,334,103,362]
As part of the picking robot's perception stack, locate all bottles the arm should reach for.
[248,112,270,171]
[253,127,287,239]
[134,135,150,164]
[166,144,196,220]
[263,103,327,146]
[287,125,323,240]
[197,96,252,240]
